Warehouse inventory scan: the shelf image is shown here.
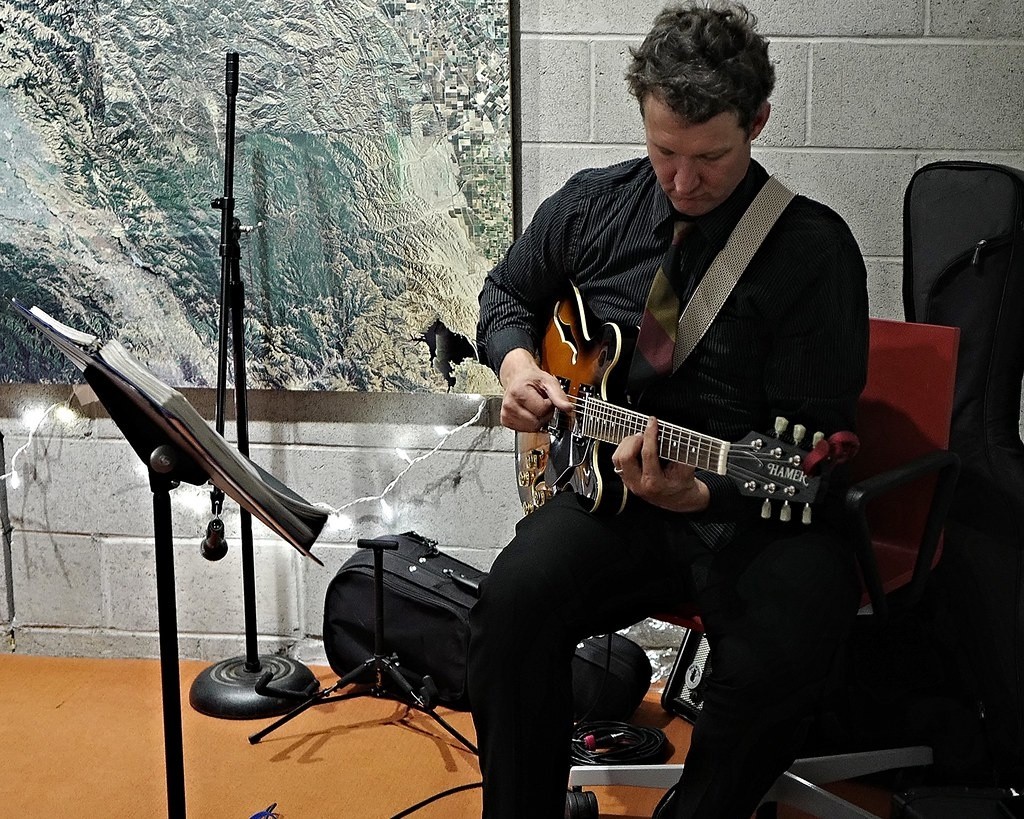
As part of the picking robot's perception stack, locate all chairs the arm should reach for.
[561,315,960,817]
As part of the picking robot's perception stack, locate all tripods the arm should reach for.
[247,541,479,759]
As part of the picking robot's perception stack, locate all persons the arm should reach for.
[467,1,871,819]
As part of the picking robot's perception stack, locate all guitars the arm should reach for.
[513,277,827,523]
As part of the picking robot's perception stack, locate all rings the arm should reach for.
[613,467,626,475]
